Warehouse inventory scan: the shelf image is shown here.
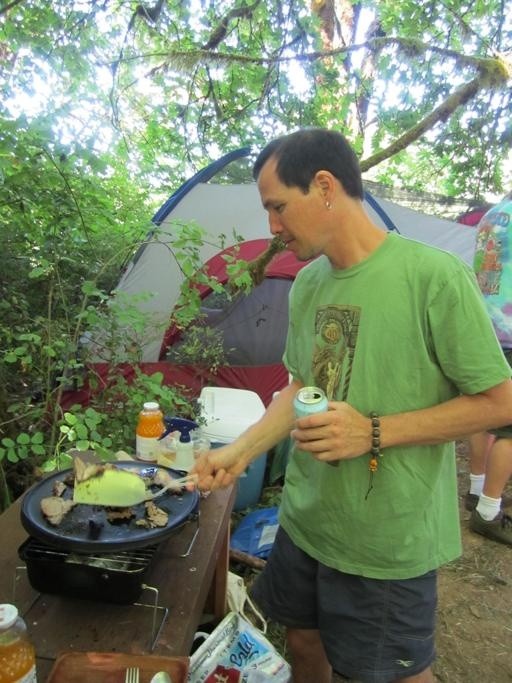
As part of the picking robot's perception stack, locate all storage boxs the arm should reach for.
[195,385,269,511]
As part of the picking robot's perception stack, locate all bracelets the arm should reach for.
[364,412,380,501]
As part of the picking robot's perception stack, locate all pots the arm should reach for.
[18,462,201,550]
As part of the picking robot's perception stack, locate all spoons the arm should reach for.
[150,670,171,683]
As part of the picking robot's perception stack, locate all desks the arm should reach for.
[1,474,245,683]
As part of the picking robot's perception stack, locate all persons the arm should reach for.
[465,191,512,545]
[184,128,512,682]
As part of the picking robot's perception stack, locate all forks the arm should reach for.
[125,666,139,683]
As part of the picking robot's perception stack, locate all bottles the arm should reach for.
[0,603,38,683]
[134,401,166,463]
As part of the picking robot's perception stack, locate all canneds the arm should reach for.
[292,385,330,425]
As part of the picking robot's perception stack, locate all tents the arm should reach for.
[29,144,475,442]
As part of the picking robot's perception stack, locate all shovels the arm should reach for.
[73,468,199,507]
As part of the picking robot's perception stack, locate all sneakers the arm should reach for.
[469,507,512,546]
[465,490,511,511]
